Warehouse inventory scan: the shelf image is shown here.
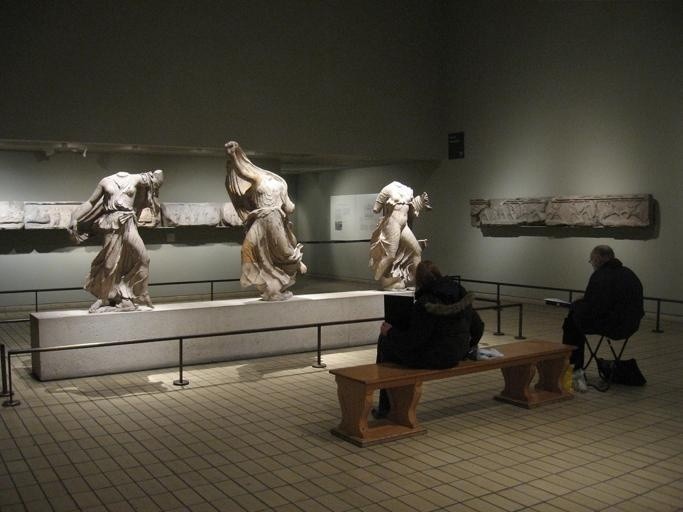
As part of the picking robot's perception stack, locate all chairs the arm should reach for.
[584,304,646,391]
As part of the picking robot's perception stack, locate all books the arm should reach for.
[384,295,414,331]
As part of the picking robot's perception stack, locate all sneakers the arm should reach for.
[371,408,385,420]
[573,370,589,392]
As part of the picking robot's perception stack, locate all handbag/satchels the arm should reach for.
[596,356,647,388]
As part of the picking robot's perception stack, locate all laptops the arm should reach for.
[383,295,414,325]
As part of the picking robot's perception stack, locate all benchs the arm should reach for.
[328,340,580,450]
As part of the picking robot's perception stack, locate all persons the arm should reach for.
[371,183,432,291]
[563,245,645,380]
[68,169,164,312]
[223,140,308,300]
[371,259,485,418]
